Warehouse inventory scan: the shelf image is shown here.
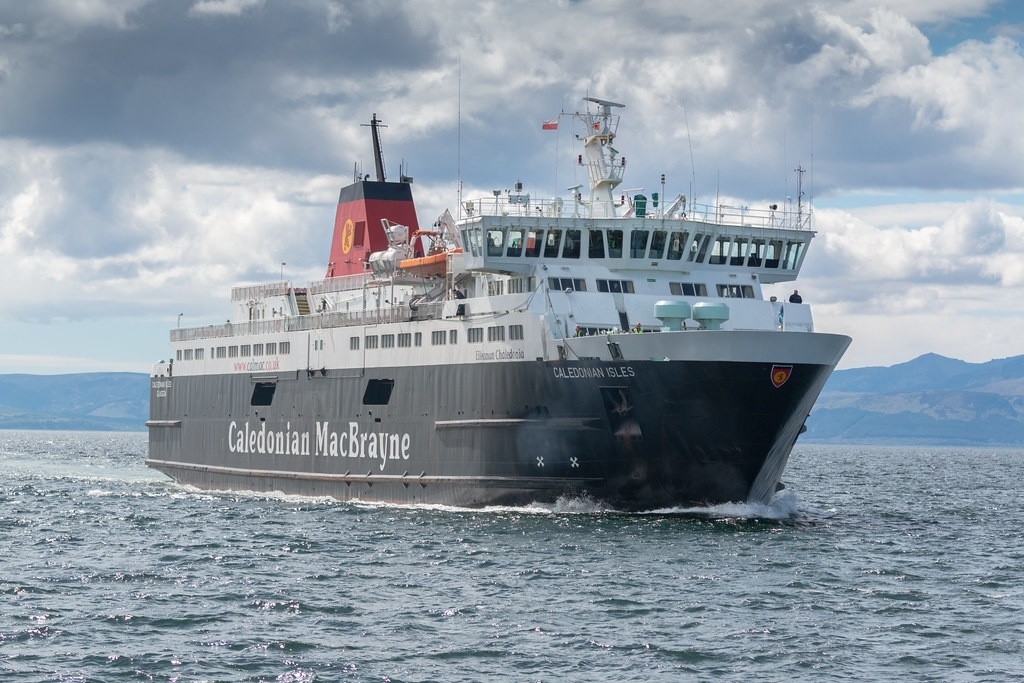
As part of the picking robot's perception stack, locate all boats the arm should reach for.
[142,53,853,510]
[400,229,463,278]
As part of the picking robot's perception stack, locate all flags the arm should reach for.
[591,121,600,130]
[542,119,558,130]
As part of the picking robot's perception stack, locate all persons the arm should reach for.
[789,290,802,304]
[573,325,584,337]
[487,233,494,247]
[453,290,466,315]
[632,322,644,334]
